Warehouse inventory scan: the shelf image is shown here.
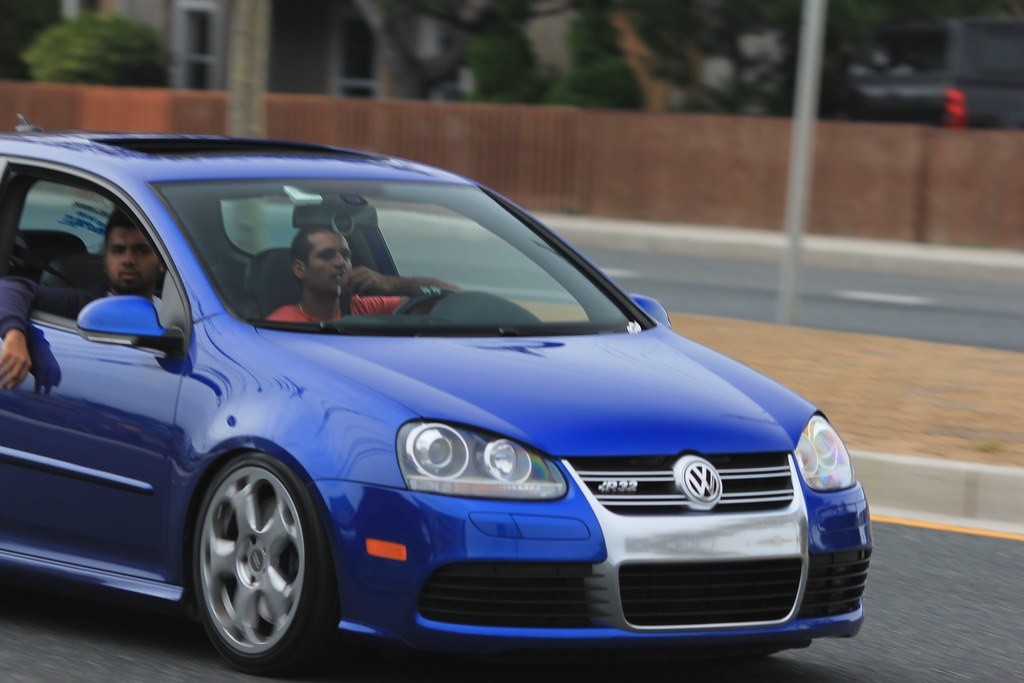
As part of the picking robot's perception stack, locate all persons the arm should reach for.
[0,205,167,393]
[264,225,461,324]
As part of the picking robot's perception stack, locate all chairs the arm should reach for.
[248,245,305,311]
[11,230,88,277]
[38,254,112,293]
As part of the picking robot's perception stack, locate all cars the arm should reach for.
[0,131,872,678]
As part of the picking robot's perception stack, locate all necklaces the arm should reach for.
[298,302,305,313]
